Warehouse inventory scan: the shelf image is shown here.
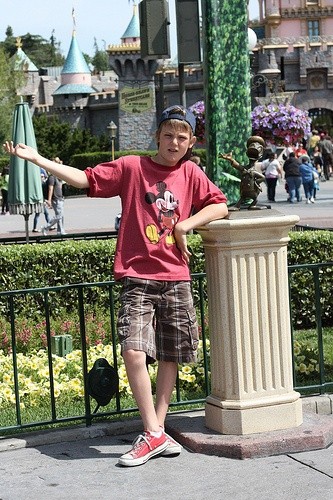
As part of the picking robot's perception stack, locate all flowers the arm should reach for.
[251,102,313,146]
[188,99,206,145]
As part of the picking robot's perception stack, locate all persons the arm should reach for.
[277,128,333,193]
[40,156,69,236]
[300,157,316,203]
[309,160,319,200]
[0,166,11,214]
[261,152,282,202]
[2,105,228,468]
[190,151,204,172]
[283,151,303,203]
[32,172,58,233]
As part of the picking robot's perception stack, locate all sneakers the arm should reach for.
[117,431,170,466]
[158,426,183,455]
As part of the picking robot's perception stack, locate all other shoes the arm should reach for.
[311,199,315,203]
[41,227,48,236]
[306,200,310,203]
[49,227,57,230]
[32,229,41,232]
[57,232,67,235]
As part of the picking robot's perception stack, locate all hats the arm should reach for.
[158,104,196,160]
[320,133,325,138]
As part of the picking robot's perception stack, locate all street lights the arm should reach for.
[107,121,117,161]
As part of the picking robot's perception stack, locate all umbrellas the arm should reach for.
[7,97,47,242]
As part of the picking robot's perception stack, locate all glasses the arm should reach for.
[41,175,46,179]
